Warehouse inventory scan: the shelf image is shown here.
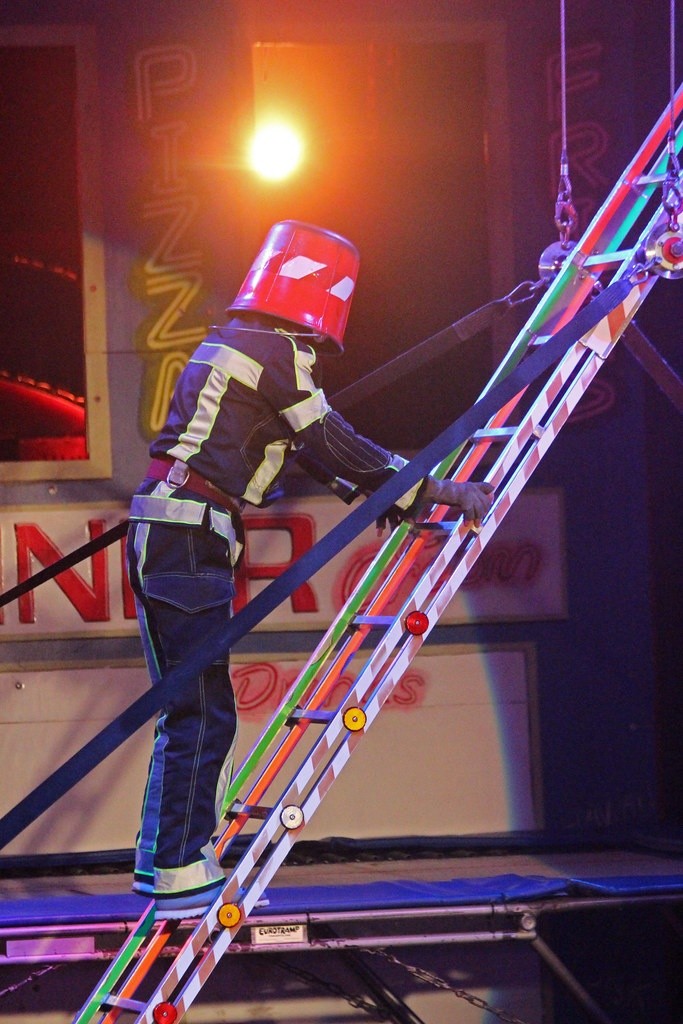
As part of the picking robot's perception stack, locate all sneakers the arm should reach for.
[129,881,155,898]
[154,886,269,919]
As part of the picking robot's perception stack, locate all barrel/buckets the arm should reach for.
[209,220,360,357]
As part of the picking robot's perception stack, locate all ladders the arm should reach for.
[74,83,683,1024]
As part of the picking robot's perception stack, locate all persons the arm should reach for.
[127,221,494,921]
